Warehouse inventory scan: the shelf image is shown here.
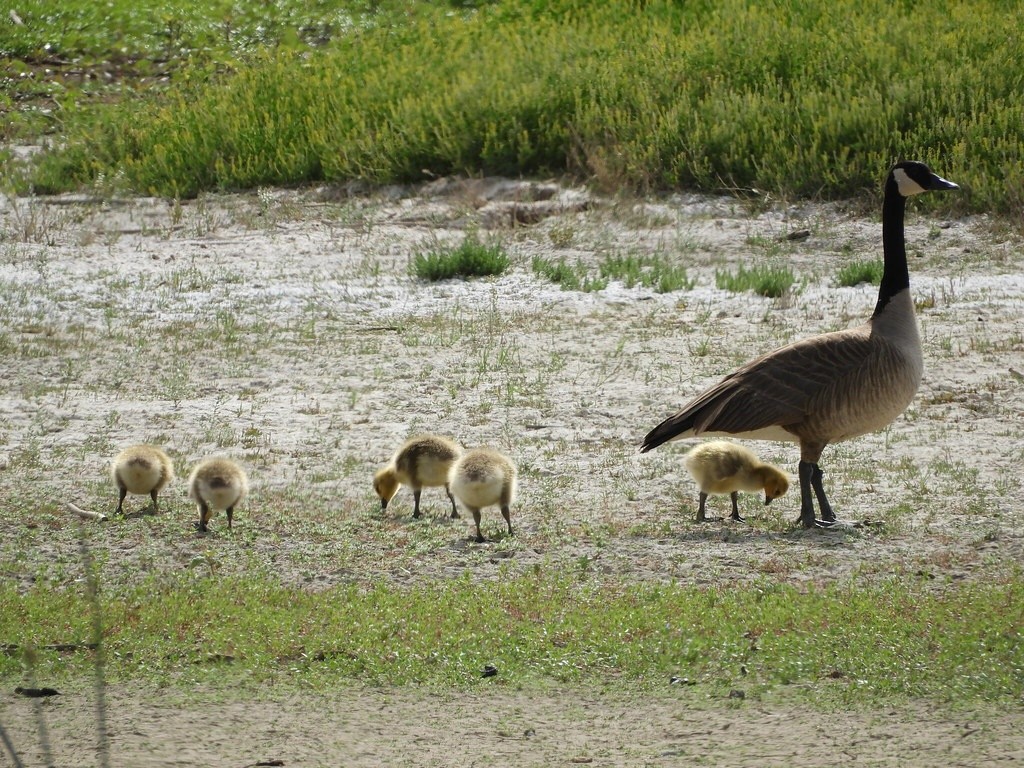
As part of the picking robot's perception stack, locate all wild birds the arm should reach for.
[448,449,517,543]
[686,440,788,523]
[189,456,248,532]
[110,446,173,514]
[638,160,960,529]
[372,435,462,520]
